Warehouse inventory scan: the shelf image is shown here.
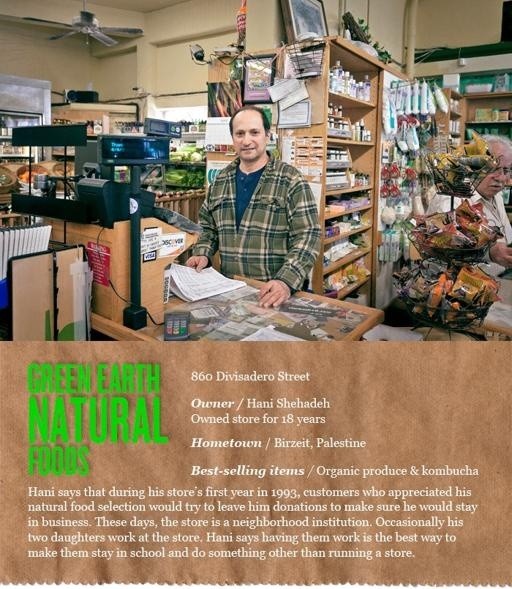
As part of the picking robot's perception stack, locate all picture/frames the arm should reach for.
[280,0,329,44]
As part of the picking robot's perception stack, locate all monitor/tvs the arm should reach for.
[74,139,114,191]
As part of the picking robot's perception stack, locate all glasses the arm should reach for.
[491,167,511,179]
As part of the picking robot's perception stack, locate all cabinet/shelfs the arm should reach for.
[433,87,511,149]
[207,39,385,311]
[0,109,44,166]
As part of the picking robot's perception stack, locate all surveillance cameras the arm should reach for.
[190,43,204,61]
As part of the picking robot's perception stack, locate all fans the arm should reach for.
[21,0,146,49]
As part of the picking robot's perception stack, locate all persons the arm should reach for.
[426,136,512,277]
[185,107,322,308]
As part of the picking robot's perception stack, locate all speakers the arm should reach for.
[76,90,99,102]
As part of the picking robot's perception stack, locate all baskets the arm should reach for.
[407,228,496,269]
[398,273,494,332]
[0,161,75,194]
[280,39,325,81]
[421,147,499,200]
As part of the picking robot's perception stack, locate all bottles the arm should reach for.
[326,59,372,189]
